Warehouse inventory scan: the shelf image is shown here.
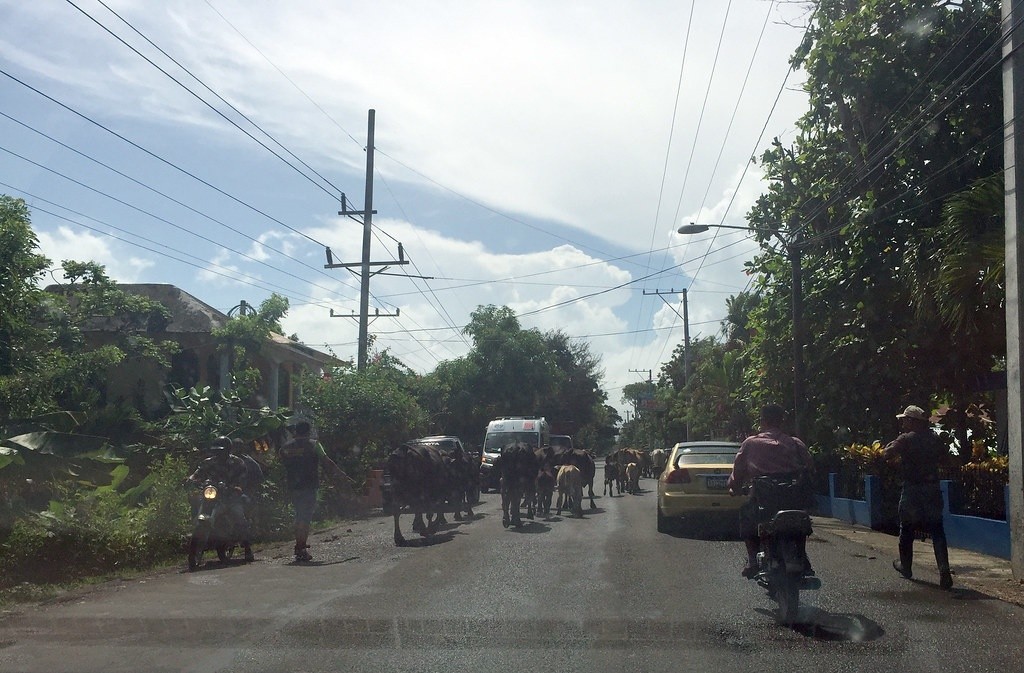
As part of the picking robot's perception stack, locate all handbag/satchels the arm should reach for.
[751,470,818,520]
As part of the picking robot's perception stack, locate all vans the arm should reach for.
[479,416,549,489]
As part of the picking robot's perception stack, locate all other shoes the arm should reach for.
[741,563,759,577]
[893,559,912,579]
[296,552,312,560]
[939,574,953,591]
[244,548,254,562]
[295,545,298,555]
[797,555,812,569]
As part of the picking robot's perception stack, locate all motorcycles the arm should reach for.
[174,478,244,572]
[729,487,822,627]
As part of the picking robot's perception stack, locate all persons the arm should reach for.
[189,436,263,563]
[882,405,954,589]
[278,420,346,561]
[728,403,815,577]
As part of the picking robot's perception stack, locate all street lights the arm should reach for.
[678,223,804,451]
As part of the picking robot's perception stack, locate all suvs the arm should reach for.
[548,434,574,454]
[379,436,480,511]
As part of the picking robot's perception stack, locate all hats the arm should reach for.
[895,405,926,421]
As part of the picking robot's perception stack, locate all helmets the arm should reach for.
[210,436,233,451]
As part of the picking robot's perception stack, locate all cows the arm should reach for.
[389,441,478,545]
[603,447,665,497]
[493,440,597,528]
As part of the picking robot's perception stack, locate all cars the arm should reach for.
[657,441,746,534]
